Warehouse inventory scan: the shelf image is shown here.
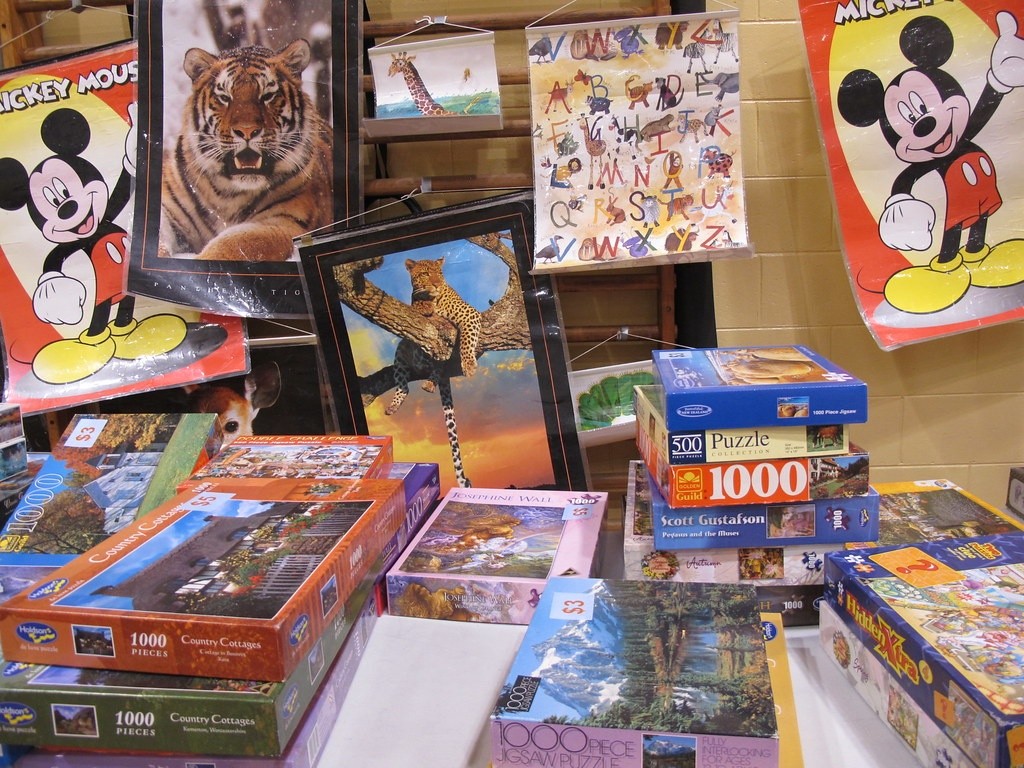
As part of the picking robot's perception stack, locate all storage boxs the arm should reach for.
[176,435,394,497]
[0,402,50,523]
[824,533,1024,768]
[867,476,1024,551]
[0,552,386,759]
[756,585,824,627]
[0,584,377,768]
[374,462,441,586]
[652,345,868,431]
[633,384,850,466]
[0,479,410,682]
[647,464,881,551]
[0,566,63,608]
[1006,466,1024,519]
[384,487,610,624]
[634,418,869,510]
[624,460,847,586]
[757,612,805,768]
[490,577,779,768]
[818,601,977,768]
[0,412,224,565]
[370,500,441,617]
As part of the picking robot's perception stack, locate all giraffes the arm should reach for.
[388,51,461,116]
